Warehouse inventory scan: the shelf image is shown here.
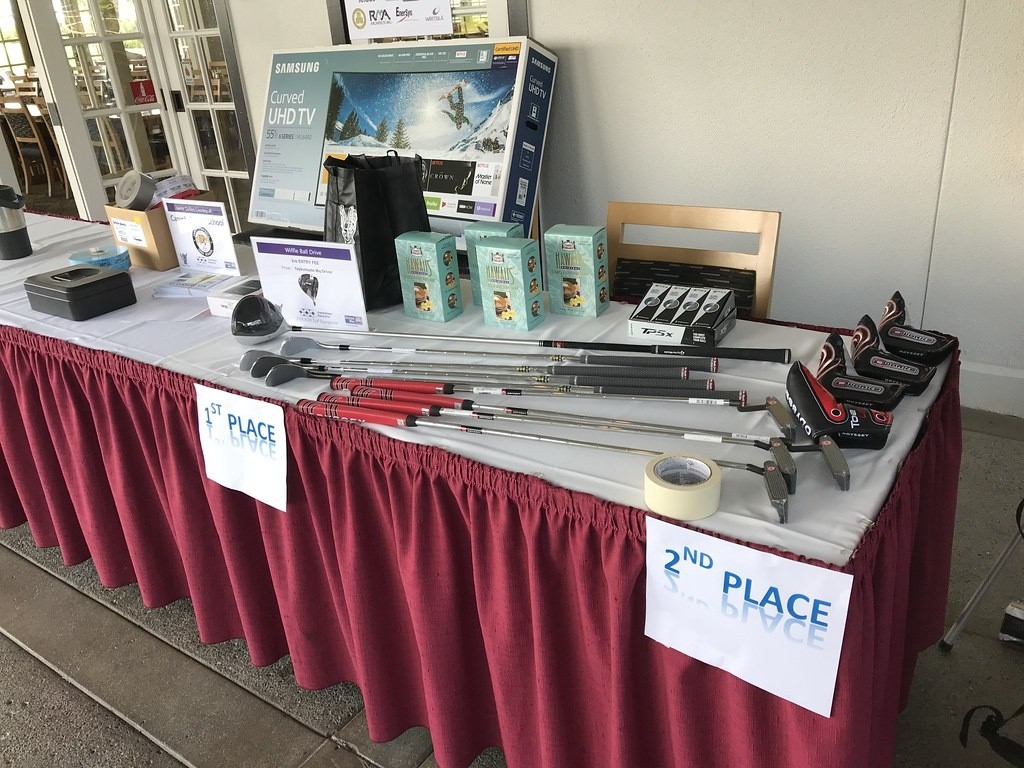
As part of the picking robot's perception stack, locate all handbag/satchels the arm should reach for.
[323,150,431,312]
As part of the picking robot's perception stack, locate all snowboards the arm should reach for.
[437,79,468,102]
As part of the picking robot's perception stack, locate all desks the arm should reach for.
[1,213,960,768]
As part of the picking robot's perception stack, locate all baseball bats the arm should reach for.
[279,336,719,374]
[331,376,797,443]
[264,363,748,405]
[295,396,789,525]
[350,385,851,495]
[239,350,689,381]
[316,391,798,494]
[231,293,794,365]
[250,355,715,391]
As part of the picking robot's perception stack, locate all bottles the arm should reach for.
[139,82,146,96]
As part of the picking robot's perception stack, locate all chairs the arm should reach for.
[0,58,230,198]
[605,202,781,320]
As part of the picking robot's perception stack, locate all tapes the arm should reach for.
[114,169,156,211]
[144,174,201,212]
[644,451,721,522]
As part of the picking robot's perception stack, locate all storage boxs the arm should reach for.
[629,308,737,347]
[630,283,672,324]
[691,289,736,329]
[106,189,213,272]
[544,224,610,319]
[651,286,691,325]
[475,235,545,332]
[464,220,524,308]
[395,231,464,323]
[247,35,558,255]
[207,275,263,318]
[670,288,710,326]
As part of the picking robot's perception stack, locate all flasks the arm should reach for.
[0,185,33,260]
